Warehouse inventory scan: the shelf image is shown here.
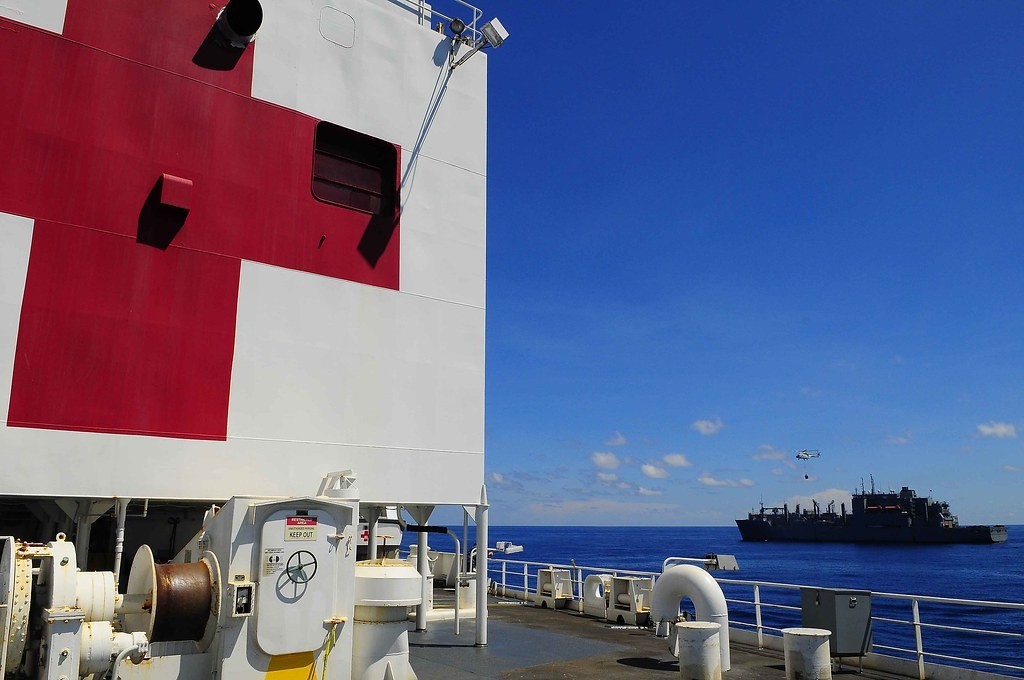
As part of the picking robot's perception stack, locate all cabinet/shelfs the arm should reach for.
[799,585,873,675]
[428,552,464,587]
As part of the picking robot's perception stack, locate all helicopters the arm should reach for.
[795,448,822,461]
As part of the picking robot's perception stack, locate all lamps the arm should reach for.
[705,552,740,572]
[449,17,509,71]
[504,542,523,554]
[450,18,465,39]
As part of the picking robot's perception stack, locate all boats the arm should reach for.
[734,473,1009,544]
